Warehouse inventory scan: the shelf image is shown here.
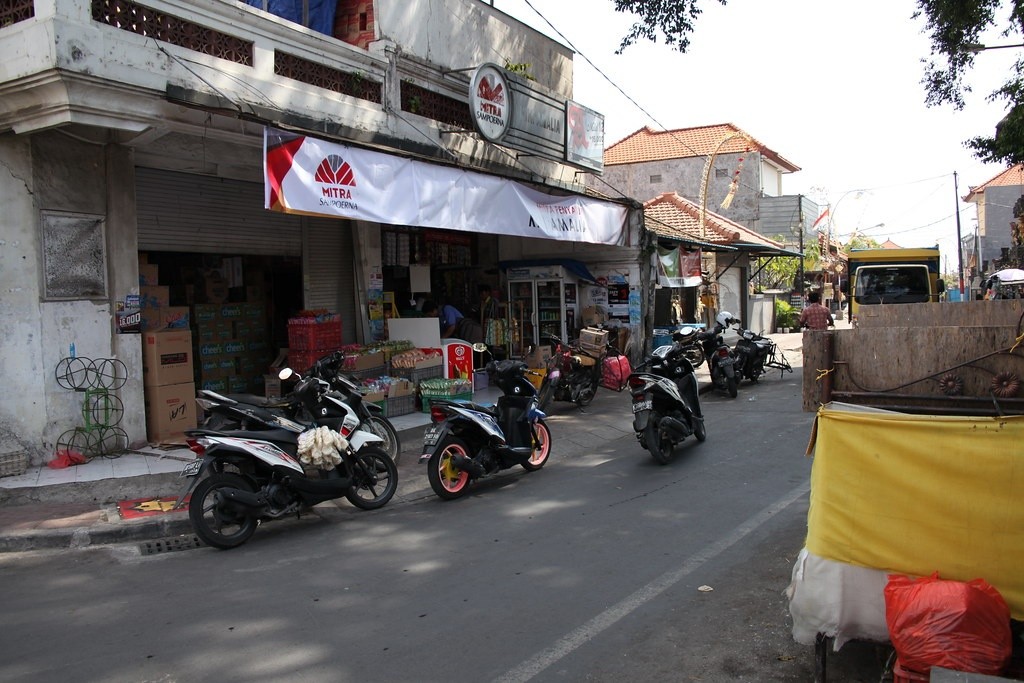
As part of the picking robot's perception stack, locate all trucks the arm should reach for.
[840,244,944,330]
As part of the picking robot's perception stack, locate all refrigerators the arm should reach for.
[506,265,579,360]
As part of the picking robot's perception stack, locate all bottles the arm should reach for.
[539,287,562,338]
[789,292,801,308]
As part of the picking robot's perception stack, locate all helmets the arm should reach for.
[716,312,732,328]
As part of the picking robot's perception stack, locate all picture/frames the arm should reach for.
[43,210,107,303]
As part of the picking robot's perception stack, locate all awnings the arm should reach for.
[716,244,806,282]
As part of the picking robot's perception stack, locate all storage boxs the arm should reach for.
[579,326,609,358]
[138,253,494,440]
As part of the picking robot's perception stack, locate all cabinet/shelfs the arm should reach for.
[509,269,577,355]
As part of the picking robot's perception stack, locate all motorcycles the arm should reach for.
[171,360,399,548]
[537,330,606,413]
[670,326,705,367]
[195,350,401,473]
[731,328,771,386]
[679,322,738,398]
[626,339,707,466]
[416,341,552,500]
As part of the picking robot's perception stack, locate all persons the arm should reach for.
[798,292,833,330]
[475,284,499,367]
[421,301,464,339]
[790,265,811,309]
